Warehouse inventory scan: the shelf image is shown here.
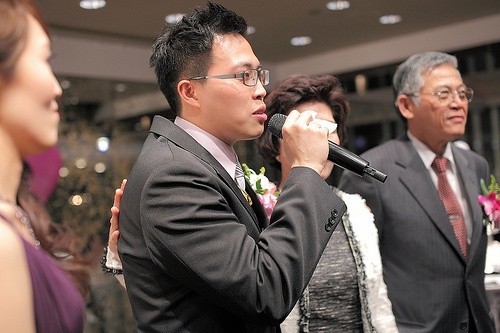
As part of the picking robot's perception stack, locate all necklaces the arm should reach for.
[1,199,42,247]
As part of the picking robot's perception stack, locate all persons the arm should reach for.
[117,2,347,333]
[0,0,89,333]
[334,53,497,333]
[101,74,400,333]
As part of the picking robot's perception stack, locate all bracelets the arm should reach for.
[101,245,124,277]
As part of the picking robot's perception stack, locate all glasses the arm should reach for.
[188,70,269,86]
[405,87,474,103]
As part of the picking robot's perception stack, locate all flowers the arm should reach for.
[241,163,282,224]
[478,174,500,236]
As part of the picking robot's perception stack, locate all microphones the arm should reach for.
[268,114,387,183]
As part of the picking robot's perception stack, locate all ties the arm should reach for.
[235,161,245,196]
[433,157,468,261]
